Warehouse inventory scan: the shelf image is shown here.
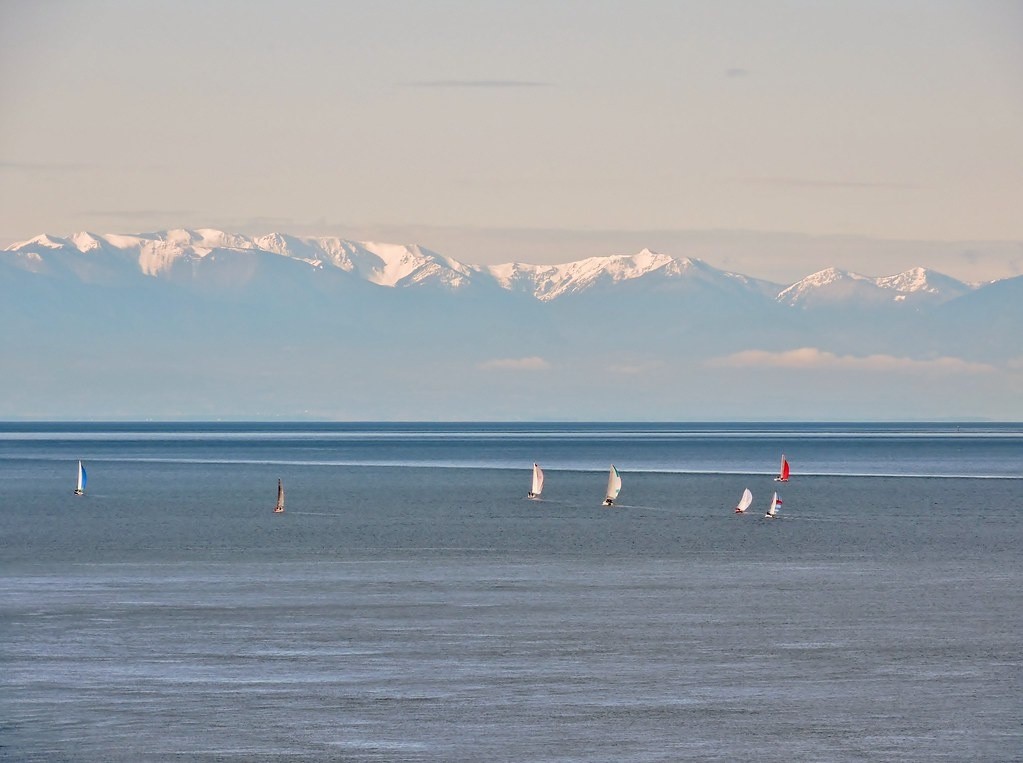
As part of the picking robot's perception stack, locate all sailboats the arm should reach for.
[773,454,790,482]
[734,487,753,513]
[73,460,87,495]
[764,491,783,518]
[527,461,543,499]
[602,464,621,506]
[273,478,285,513]
